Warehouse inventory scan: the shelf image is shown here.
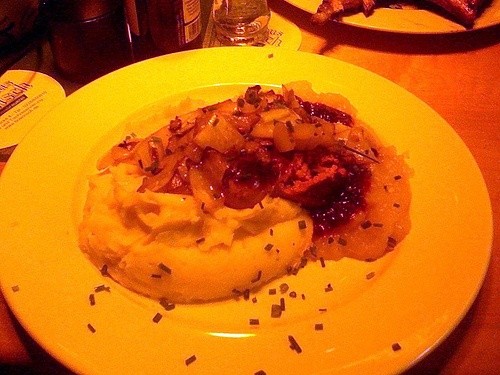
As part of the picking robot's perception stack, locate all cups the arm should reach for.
[211,0,270,46]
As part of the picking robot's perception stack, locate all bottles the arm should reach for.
[123,0,218,61]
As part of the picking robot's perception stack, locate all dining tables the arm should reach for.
[1,0,498,375]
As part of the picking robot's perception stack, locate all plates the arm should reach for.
[0,45,496,374]
[286,0,499,36]
